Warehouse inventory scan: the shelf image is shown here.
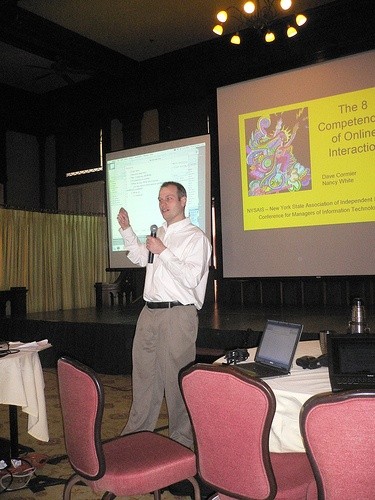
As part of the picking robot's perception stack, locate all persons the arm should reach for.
[117,182,213,454]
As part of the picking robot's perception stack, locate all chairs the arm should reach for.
[299,389,375,500]
[57,355,201,500]
[178,360,319,500]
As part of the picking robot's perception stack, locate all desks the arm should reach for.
[0,341,52,475]
[212,339,332,453]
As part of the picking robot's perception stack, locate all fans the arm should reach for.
[27,56,97,86]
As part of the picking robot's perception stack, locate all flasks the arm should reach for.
[351,297,366,333]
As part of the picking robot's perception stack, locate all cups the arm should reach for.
[320,330,332,355]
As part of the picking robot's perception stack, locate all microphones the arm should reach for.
[148,225,158,264]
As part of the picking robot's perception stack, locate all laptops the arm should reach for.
[237,319,304,379]
[326,333,375,394]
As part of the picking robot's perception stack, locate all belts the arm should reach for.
[146,301,194,309]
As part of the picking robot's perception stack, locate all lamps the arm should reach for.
[213,0,307,45]
